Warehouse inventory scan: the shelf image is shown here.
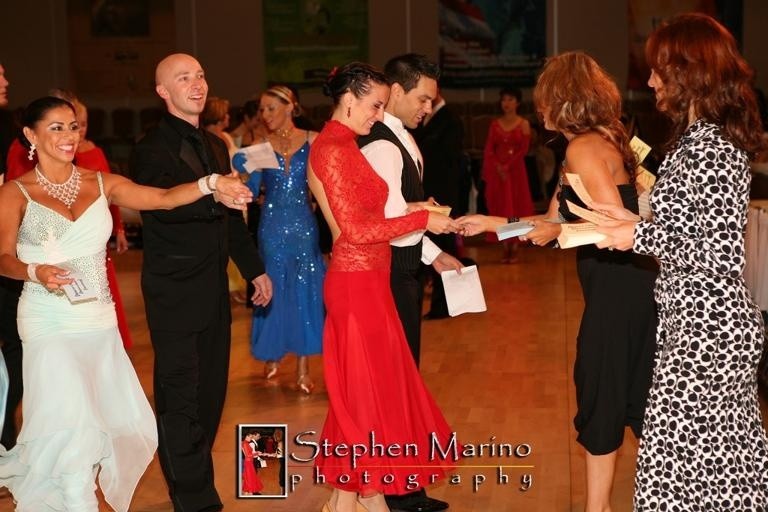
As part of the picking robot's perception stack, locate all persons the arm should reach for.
[0,11,768,512]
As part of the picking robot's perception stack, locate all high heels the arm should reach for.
[296,374,315,395]
[264,360,281,380]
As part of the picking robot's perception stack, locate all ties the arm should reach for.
[191,130,211,172]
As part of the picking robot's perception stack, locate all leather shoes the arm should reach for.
[423,311,449,320]
[391,497,451,512]
